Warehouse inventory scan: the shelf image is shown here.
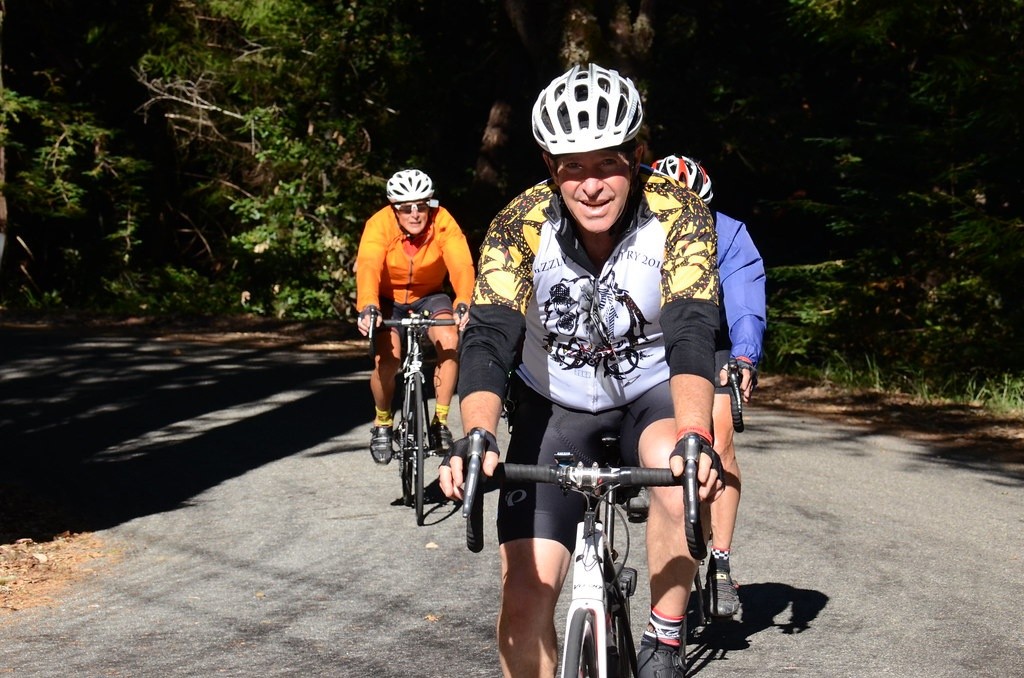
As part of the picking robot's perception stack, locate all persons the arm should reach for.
[356,168,476,465]
[437,63,726,678]
[645,154,766,615]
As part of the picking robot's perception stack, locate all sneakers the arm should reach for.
[703,572,740,616]
[427,423,453,456]
[638,647,686,678]
[369,426,393,465]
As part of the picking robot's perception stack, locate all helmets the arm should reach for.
[532,62,643,155]
[387,169,434,206]
[650,153,714,207]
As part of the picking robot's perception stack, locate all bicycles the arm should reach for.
[623,354,746,627]
[367,302,469,524]
[459,422,713,678]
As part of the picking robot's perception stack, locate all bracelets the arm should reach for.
[672,425,714,445]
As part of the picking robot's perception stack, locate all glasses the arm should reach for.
[393,202,430,212]
